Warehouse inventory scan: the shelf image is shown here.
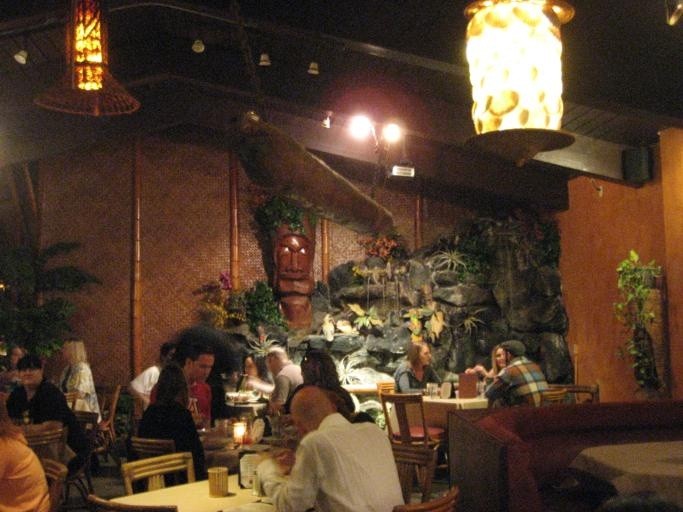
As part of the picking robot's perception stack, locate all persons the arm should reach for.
[484,339,549,408]
[463,341,505,384]
[0,339,101,512]
[389,339,448,485]
[131,325,406,512]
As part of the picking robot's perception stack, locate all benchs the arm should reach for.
[475,397,682,512]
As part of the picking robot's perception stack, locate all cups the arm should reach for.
[427,383,438,399]
[208,466,229,497]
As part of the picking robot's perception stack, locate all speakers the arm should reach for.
[623,146,651,187]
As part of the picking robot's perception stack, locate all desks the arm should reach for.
[565,439,682,512]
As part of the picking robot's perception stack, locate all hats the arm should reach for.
[500,340,526,356]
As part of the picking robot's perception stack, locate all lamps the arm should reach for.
[32,0,143,118]
[347,114,403,165]
[190,37,206,54]
[461,0,577,166]
[322,111,334,131]
[307,62,321,76]
[259,52,271,67]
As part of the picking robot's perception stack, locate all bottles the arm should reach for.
[238,412,258,490]
